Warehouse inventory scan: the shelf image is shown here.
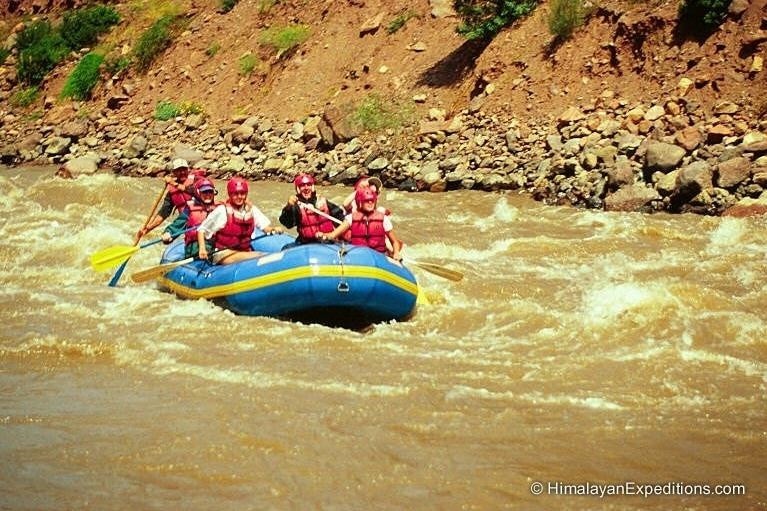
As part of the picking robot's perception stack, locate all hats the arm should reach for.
[171,158,188,171]
[198,186,214,192]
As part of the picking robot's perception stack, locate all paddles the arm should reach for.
[295,200,417,261]
[109,183,169,286]
[90,224,202,271]
[130,231,274,284]
[318,232,464,281]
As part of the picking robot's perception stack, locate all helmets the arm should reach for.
[354,177,377,196]
[192,177,214,198]
[355,186,376,211]
[227,177,249,200]
[294,174,315,195]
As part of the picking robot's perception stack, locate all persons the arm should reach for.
[136,158,205,244]
[161,178,226,259]
[342,176,392,217]
[197,177,283,265]
[279,175,352,251]
[314,187,400,261]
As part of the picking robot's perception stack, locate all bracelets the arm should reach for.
[175,182,179,188]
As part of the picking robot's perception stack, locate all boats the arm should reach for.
[153,228,420,326]
